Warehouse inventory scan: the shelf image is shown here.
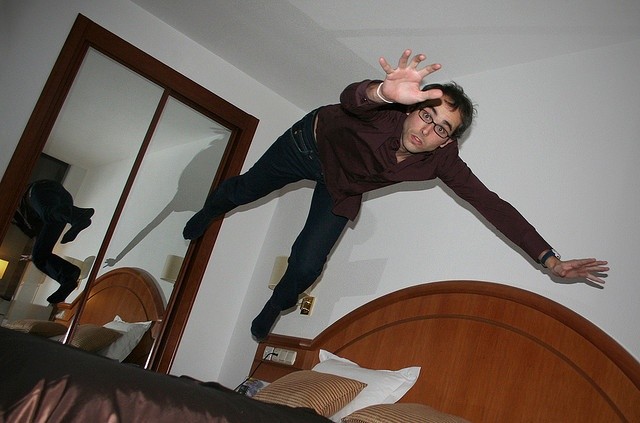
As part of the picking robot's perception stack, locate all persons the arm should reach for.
[11,178,94,303]
[183,50,609,341]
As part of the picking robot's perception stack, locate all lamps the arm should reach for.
[65,256,85,290]
[160,253,184,284]
[267,256,315,316]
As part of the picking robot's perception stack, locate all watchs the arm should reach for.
[540,248,561,268]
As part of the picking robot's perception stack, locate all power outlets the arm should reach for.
[262,346,274,360]
[272,347,282,362]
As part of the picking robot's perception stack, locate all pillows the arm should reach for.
[2,320,68,337]
[311,348,422,423]
[251,368,368,417]
[95,314,152,362]
[341,403,470,423]
[49,323,124,352]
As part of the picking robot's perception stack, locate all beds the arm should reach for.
[0,266,169,369]
[1,280,639,423]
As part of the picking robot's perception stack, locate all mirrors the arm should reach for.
[0,12,260,376]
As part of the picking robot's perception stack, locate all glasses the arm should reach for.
[416,106,451,139]
